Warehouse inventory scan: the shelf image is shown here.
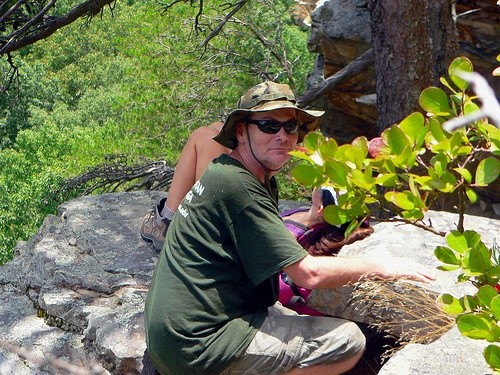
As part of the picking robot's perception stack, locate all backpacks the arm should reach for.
[278,215,369,315]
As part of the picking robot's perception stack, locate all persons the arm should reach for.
[144,80,435,375]
[141,121,374,316]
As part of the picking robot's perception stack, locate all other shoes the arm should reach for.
[141,348,160,375]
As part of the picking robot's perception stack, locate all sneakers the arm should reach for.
[140,198,172,254]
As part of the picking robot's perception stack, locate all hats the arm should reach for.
[212,80,325,150]
[309,153,361,229]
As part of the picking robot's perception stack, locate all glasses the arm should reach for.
[242,117,303,135]
